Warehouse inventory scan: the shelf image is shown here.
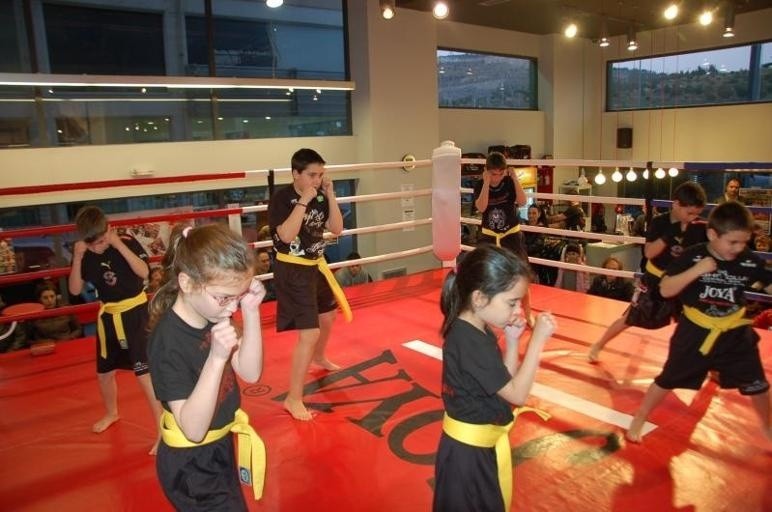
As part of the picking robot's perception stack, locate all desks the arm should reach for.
[4,303,45,316]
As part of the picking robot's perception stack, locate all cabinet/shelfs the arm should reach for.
[545,235,643,292]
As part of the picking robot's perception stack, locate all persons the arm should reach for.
[146,224,266,507]
[270,148,355,420]
[27,287,86,346]
[586,258,639,303]
[712,178,754,206]
[516,203,548,263]
[554,243,589,293]
[432,245,558,507]
[470,153,536,330]
[751,234,772,293]
[632,201,663,236]
[625,201,772,446]
[586,181,708,364]
[549,188,586,245]
[70,205,165,455]
[334,251,371,287]
[252,248,277,303]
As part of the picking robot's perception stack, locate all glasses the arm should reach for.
[199,282,250,307]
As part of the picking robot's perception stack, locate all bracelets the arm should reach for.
[293,201,309,208]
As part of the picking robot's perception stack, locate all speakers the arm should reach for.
[616,127,633,149]
